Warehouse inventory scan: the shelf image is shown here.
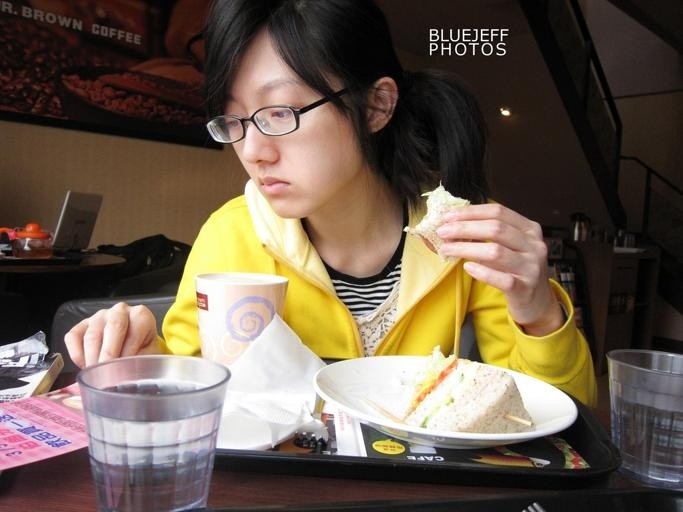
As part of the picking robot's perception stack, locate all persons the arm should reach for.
[64,1,598,411]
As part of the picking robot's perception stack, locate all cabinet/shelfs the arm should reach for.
[546,224,661,376]
[542,231,598,365]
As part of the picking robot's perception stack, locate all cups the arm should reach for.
[592,223,640,248]
[605,343,681,486]
[195,267,288,415]
[83,356,232,512]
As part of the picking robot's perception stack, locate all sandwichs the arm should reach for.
[390,344,533,434]
[403,179,471,263]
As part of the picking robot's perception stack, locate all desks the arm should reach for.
[0,370,683,512]
[0,251,127,276]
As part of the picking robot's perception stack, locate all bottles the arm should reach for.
[608,286,636,314]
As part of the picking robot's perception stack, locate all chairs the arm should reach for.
[107,265,177,296]
[49,293,176,373]
[0,290,31,336]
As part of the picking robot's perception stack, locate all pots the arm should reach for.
[11,221,50,255]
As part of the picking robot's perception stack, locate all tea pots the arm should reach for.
[568,211,591,239]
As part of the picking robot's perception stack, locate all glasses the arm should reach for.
[204,77,369,145]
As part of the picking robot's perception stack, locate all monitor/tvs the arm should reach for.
[52,190,104,250]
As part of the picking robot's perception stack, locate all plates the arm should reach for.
[314,354,579,444]
[57,62,214,130]
